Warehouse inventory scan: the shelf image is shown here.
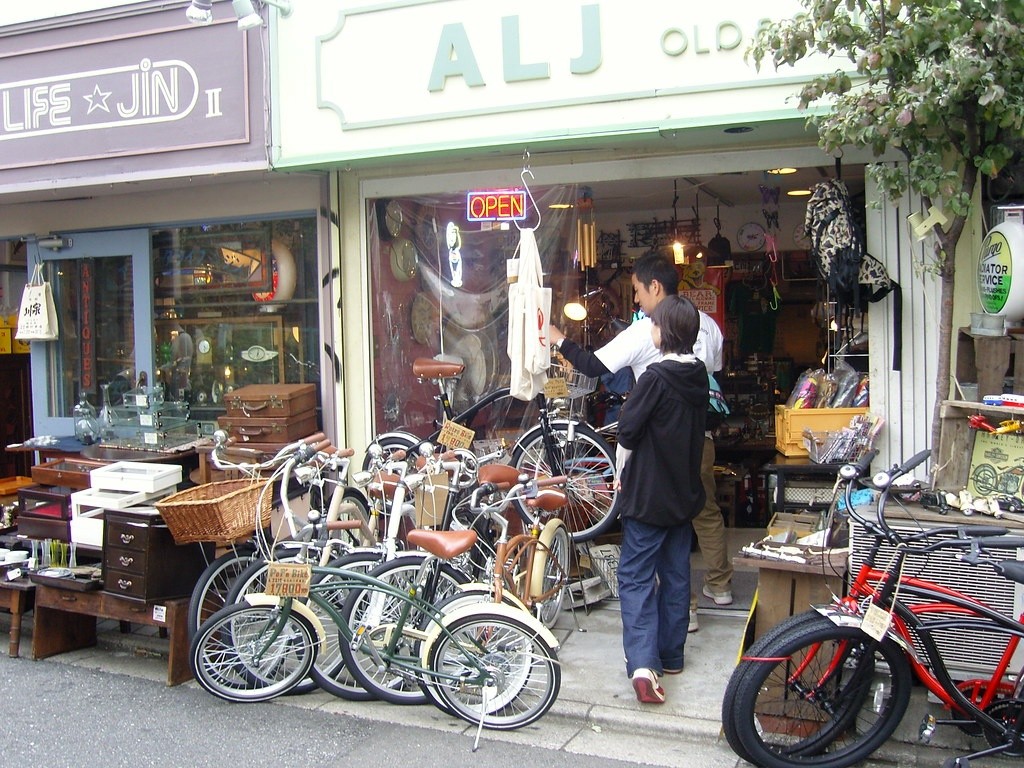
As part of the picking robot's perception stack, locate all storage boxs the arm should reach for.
[774,404,870,459]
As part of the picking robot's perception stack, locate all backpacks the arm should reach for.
[804,179,890,312]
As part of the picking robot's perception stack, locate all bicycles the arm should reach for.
[152,357,619,731]
[722,450,1024,768]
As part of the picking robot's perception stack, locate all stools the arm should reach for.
[715,468,750,528]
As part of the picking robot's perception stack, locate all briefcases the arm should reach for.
[222,383,318,418]
[217,415,317,443]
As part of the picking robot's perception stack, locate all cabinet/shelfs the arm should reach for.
[15,458,183,547]
[100,507,216,605]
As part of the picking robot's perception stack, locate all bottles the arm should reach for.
[98,385,118,438]
[76,409,98,445]
[742,419,751,441]
[73,392,96,441]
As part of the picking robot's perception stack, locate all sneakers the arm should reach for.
[702,586,733,604]
[631,668,664,703]
[686,609,698,632]
[662,667,683,673]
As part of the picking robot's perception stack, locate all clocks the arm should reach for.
[737,222,765,252]
[792,223,811,250]
[199,339,210,354]
[248,346,265,360]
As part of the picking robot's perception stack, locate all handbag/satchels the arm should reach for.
[13,262,60,342]
[702,374,729,430]
[507,228,551,401]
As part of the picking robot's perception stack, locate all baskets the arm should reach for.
[546,357,598,400]
[152,474,273,544]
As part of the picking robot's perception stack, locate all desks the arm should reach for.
[32,582,229,688]
[765,454,870,513]
[5,436,199,487]
[0,575,36,659]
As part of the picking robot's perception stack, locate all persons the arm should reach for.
[613,295,710,703]
[548,251,734,632]
